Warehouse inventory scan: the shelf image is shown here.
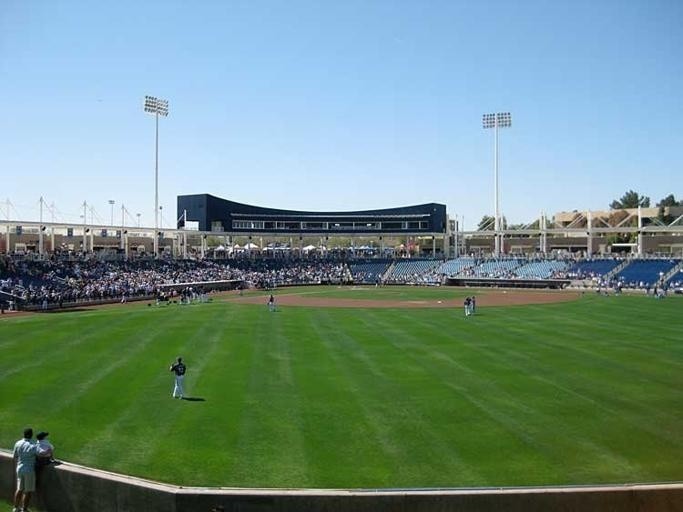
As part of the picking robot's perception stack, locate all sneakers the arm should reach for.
[13,506,29,512]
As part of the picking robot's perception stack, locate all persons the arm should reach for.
[11,429,52,512]
[0,245,682,315]
[35,431,55,466]
[168,358,186,400]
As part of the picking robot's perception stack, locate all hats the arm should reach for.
[36,432,49,440]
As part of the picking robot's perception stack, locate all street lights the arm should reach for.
[143,95,168,258]
[481,112,513,255]
